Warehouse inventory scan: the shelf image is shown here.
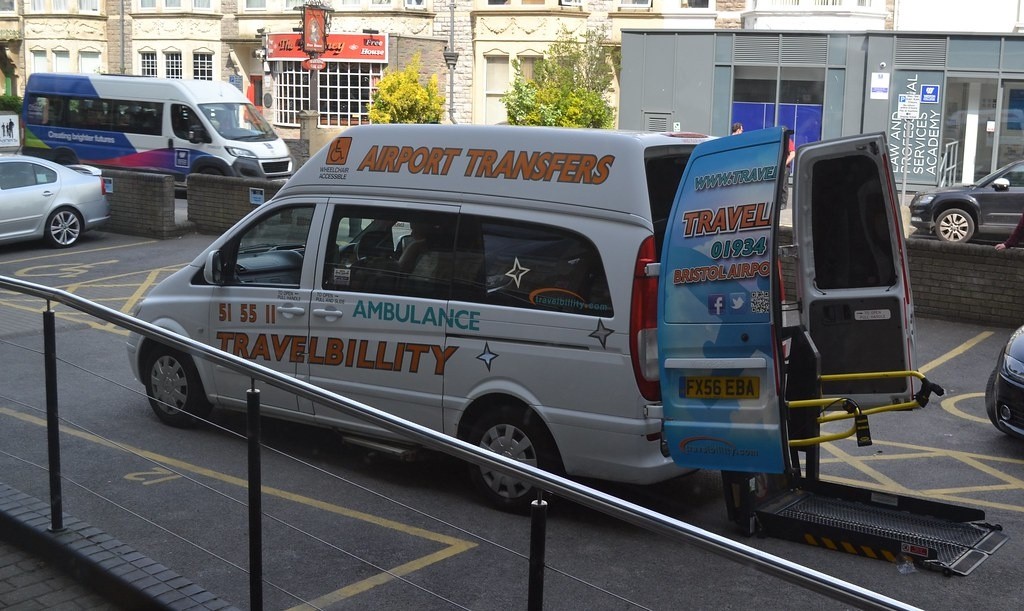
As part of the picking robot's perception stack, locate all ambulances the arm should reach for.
[119,119,918,518]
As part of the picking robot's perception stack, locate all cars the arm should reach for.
[909,158,1024,248]
[984,324,1023,443]
[0,150,111,250]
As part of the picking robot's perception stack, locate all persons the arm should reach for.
[995,209,1024,251]
[383,214,436,274]
[1,118,15,138]
[781,139,795,209]
[46,97,235,141]
[731,122,743,136]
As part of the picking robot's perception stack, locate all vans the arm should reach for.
[18,71,295,202]
